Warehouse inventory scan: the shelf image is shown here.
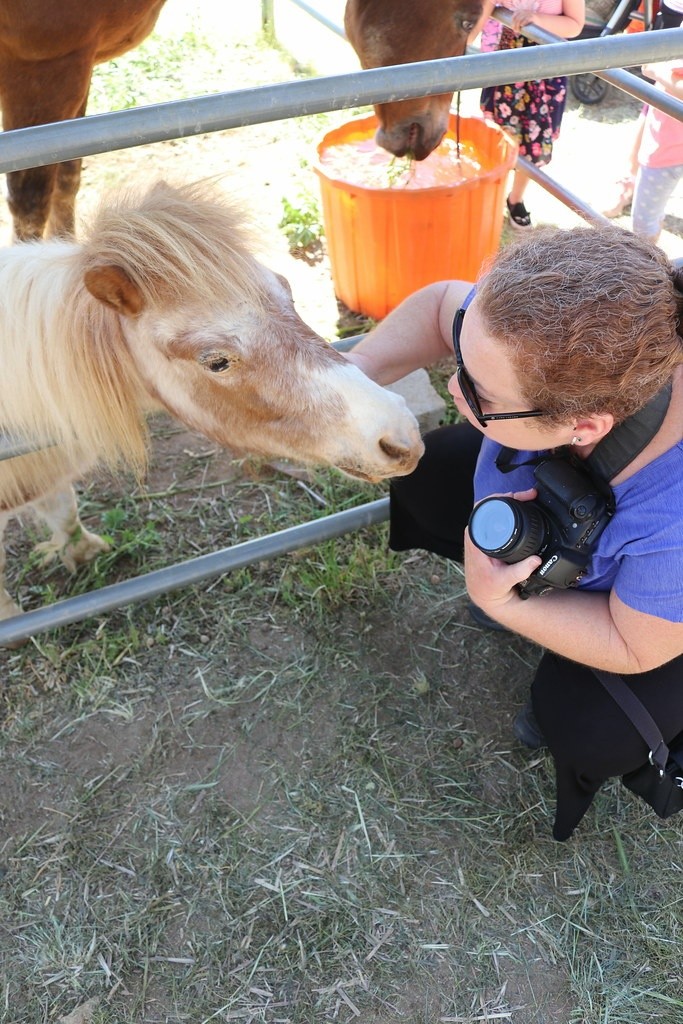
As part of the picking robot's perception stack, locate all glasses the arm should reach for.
[453,286,554,428]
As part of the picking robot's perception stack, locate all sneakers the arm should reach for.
[507,192,532,230]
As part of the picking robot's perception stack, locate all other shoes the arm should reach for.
[597,173,636,217]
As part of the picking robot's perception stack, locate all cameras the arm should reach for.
[468,459,616,599]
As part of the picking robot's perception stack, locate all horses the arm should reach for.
[0,1,482,244]
[0,173,424,654]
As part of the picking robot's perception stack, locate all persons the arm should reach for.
[344,221,683,841]
[632,59,683,244]
[661,0,683,29]
[480,0,585,231]
[602,103,649,218]
